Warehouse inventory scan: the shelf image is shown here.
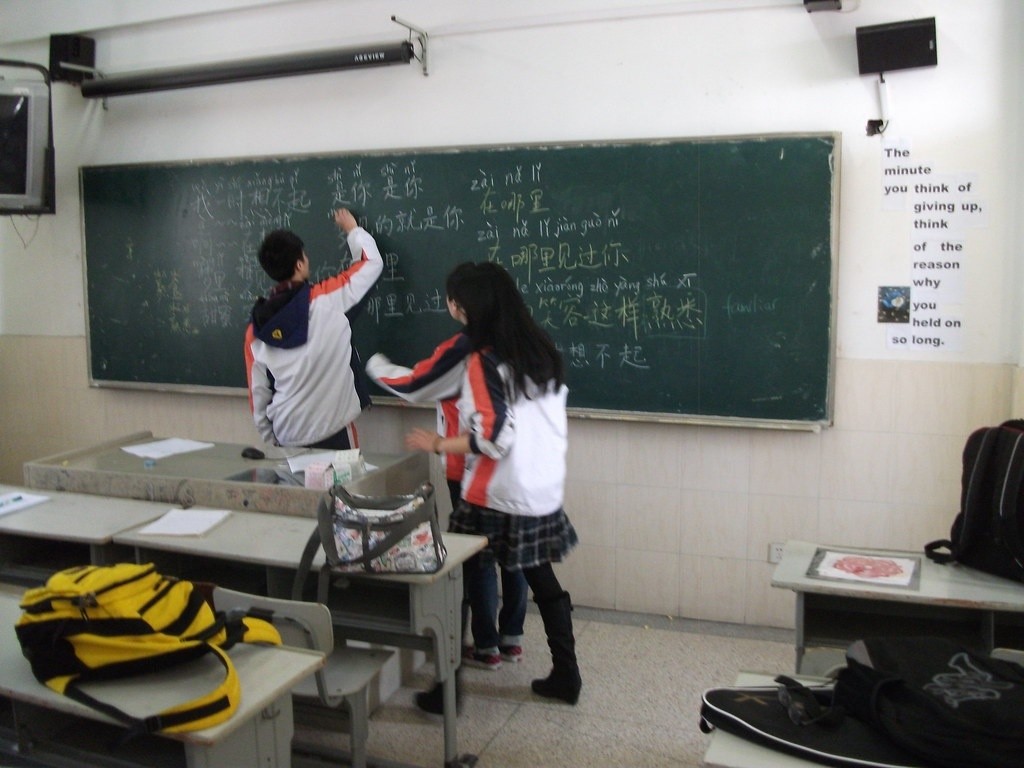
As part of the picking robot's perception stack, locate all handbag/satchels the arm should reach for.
[329,479,450,574]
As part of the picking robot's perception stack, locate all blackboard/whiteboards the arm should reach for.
[73,129,846,432]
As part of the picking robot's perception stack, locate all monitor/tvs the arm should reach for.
[0,80,49,210]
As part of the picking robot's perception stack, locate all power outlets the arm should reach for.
[767,542,785,564]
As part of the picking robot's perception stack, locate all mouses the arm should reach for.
[242,446,266,459]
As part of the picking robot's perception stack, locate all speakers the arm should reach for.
[49,34,95,84]
[856,17,938,74]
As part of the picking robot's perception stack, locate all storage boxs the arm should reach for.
[335,463,352,486]
[332,448,367,479]
[305,460,338,491]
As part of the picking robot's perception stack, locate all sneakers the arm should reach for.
[459,644,504,670]
[498,643,523,663]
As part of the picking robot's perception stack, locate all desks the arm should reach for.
[0,483,183,567]
[113,504,489,768]
[769,540,1024,675]
[0,590,327,768]
[701,667,834,768]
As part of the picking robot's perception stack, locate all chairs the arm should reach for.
[213,585,396,768]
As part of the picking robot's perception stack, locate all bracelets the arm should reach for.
[431,435,444,455]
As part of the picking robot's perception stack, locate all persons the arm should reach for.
[406,261,582,714]
[244,209,384,450]
[355,261,530,670]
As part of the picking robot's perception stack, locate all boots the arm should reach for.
[532,590,582,705]
[416,597,471,715]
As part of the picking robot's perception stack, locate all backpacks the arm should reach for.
[922,419,1024,583]
[776,632,1024,768]
[15,559,282,764]
